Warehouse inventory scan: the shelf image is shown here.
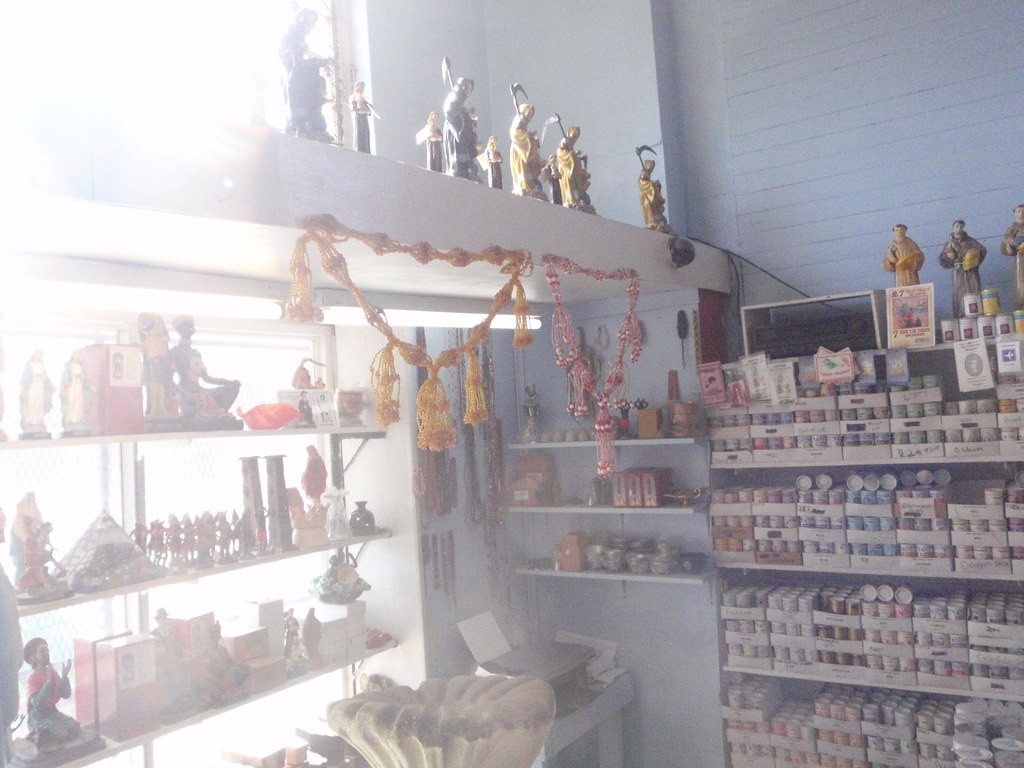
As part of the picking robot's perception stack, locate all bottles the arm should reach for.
[349,501,374,536]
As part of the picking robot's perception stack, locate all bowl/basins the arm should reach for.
[585,531,709,576]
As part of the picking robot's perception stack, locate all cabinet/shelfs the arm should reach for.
[0,423,399,768]
[703,336,1024,768]
[507,430,721,586]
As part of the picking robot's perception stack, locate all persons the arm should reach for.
[22,6,1024,755]
